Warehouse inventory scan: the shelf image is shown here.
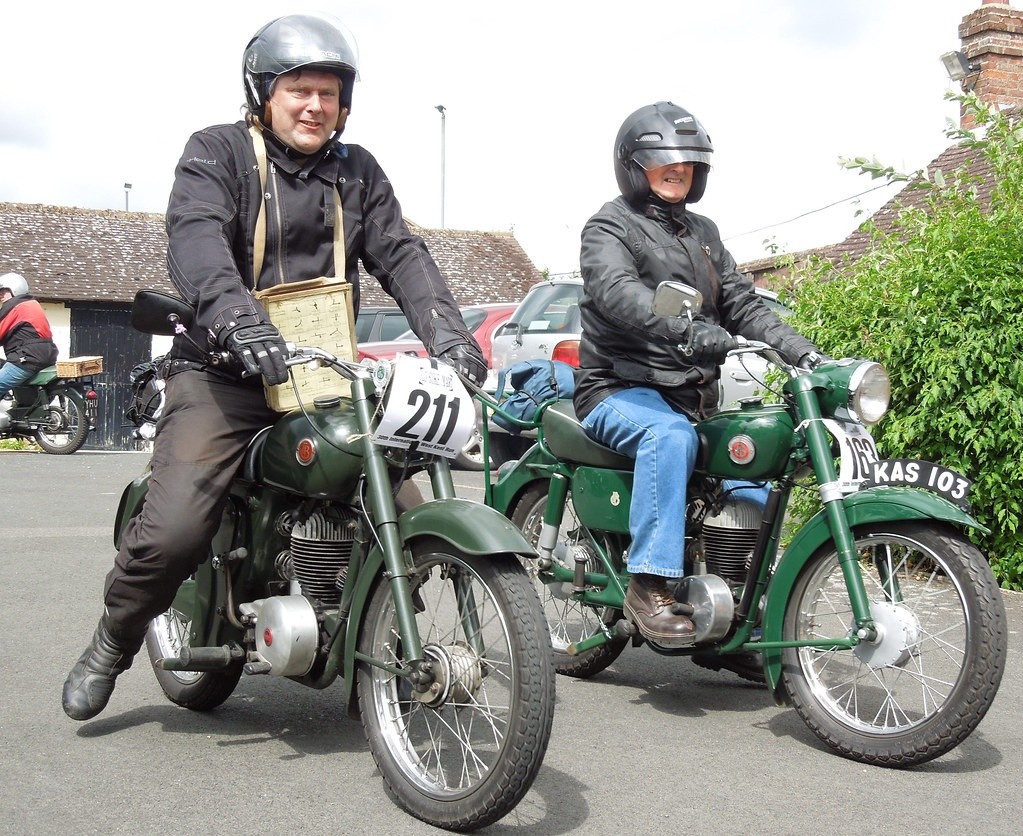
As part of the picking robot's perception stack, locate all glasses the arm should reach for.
[650,153,699,167]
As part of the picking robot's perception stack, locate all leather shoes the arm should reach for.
[691,635,772,682]
[621,573,698,644]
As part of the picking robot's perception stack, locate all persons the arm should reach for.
[573,102,830,685]
[0,273,57,401]
[63,16,488,721]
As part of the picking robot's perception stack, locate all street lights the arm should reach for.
[124,182,134,211]
[436,104,446,232]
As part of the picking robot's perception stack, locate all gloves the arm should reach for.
[686,321,736,364]
[443,343,488,394]
[226,321,290,384]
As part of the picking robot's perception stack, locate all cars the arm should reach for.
[353,307,414,344]
[353,302,571,471]
[490,280,802,371]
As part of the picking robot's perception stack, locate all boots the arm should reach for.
[62,621,130,720]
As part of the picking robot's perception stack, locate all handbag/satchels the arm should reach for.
[254,278,358,408]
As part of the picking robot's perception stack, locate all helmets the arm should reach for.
[614,100,714,206]
[243,14,353,116]
[0,273,29,298]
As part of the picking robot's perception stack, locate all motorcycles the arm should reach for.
[113,288,560,833]
[1,357,100,456]
[465,276,1009,767]
